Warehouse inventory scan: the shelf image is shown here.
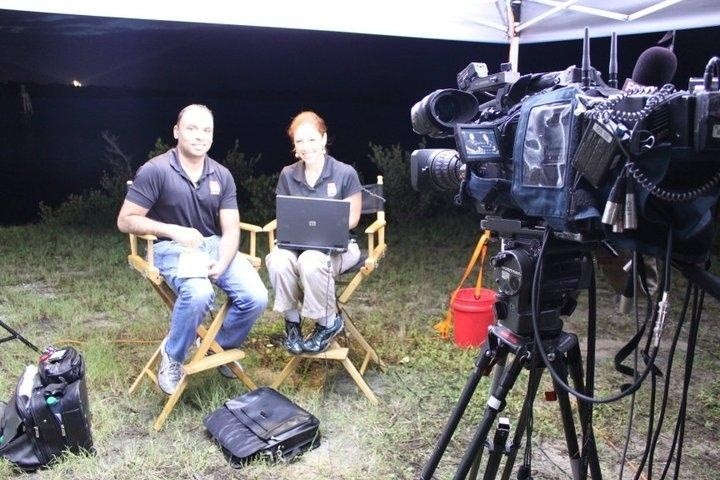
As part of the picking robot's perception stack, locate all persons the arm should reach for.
[114,104,270,395]
[264,109,364,356]
[464,133,485,155]
[482,134,498,154]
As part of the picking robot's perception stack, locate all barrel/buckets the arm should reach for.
[451,287,498,348]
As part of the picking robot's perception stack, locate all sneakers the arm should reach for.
[157,333,183,395]
[196,334,244,379]
[284,314,304,354]
[301,314,345,354]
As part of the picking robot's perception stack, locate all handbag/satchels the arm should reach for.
[37,345,85,386]
[0,364,98,475]
[201,385,322,470]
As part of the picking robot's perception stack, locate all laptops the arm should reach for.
[276,195,350,254]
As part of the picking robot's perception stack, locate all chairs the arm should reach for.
[128,181,262,432]
[262,176,387,406]
